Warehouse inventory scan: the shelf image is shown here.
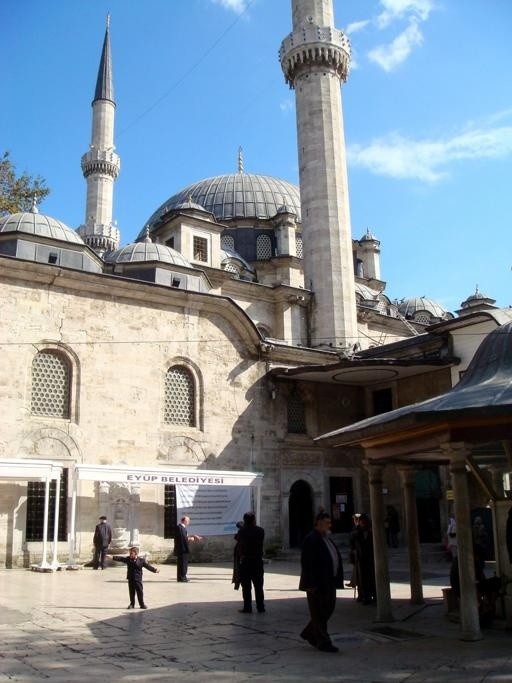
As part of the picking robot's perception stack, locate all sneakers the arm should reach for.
[300,624,340,653]
[128,604,149,609]
[237,606,253,614]
[257,607,266,614]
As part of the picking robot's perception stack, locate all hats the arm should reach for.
[98,515,107,520]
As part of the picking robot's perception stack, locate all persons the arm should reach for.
[233,512,266,612]
[384,503,401,549]
[317,506,329,514]
[105,547,160,609]
[350,513,377,605]
[471,513,490,585]
[173,516,204,582]
[345,512,363,588]
[298,512,345,652]
[92,516,112,570]
[442,516,461,601]
